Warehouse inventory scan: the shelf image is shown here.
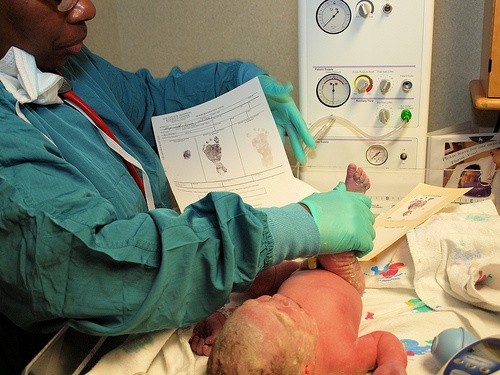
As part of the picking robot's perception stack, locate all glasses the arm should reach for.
[57,0,79,12]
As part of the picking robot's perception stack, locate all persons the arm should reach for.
[185,161,409,375]
[0,0,375,338]
[457,147,500,188]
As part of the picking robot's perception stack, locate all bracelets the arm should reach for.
[215,306,233,318]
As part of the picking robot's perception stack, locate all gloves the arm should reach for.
[298,181,376,258]
[257,76,317,166]
